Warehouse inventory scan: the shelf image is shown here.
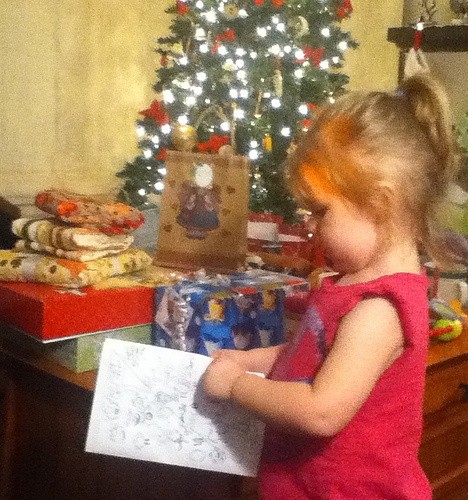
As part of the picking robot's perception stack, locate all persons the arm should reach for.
[194,71,462,500]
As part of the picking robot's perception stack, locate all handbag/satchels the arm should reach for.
[157,103,249,273]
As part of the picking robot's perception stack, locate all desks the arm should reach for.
[1,329,468,500]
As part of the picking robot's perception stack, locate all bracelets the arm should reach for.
[227,372,251,401]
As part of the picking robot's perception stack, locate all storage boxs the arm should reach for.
[1,320,152,375]
[151,268,311,362]
[0,266,187,346]
